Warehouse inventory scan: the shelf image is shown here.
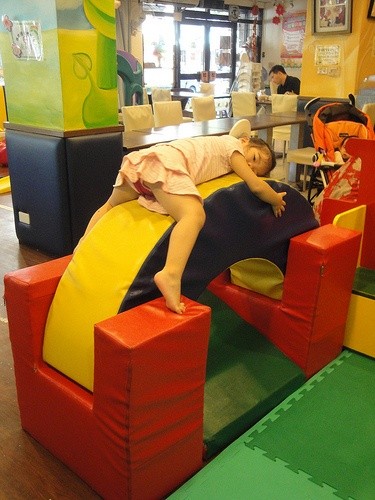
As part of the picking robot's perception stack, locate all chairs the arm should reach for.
[232,92,257,117]
[199,83,214,94]
[151,88,172,104]
[272,94,298,167]
[192,96,216,122]
[153,101,182,127]
[122,104,154,132]
[229,53,262,118]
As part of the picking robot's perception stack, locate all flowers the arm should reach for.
[272,0,295,25]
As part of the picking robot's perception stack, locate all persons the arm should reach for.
[72,133,288,315]
[334,135,359,167]
[268,65,301,95]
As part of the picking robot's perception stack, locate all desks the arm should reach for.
[147,91,230,99]
[123,112,306,182]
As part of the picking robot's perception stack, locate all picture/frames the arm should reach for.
[311,0,352,35]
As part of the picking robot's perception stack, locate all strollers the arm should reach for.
[304,94,375,205]
